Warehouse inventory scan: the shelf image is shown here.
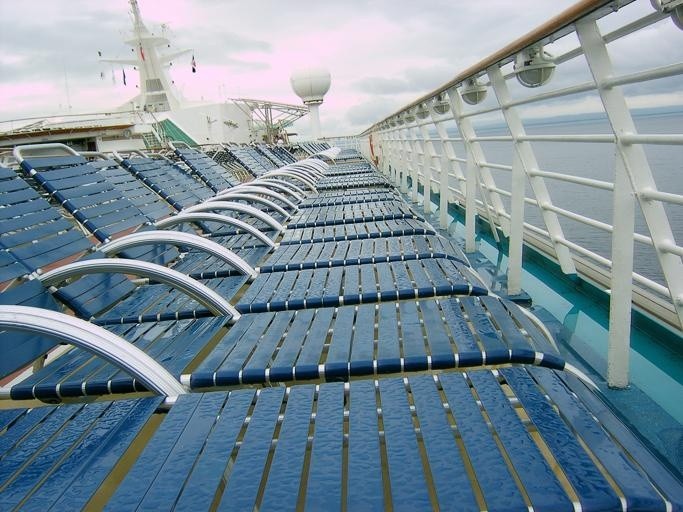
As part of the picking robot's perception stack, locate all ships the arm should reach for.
[2,0,682,507]
